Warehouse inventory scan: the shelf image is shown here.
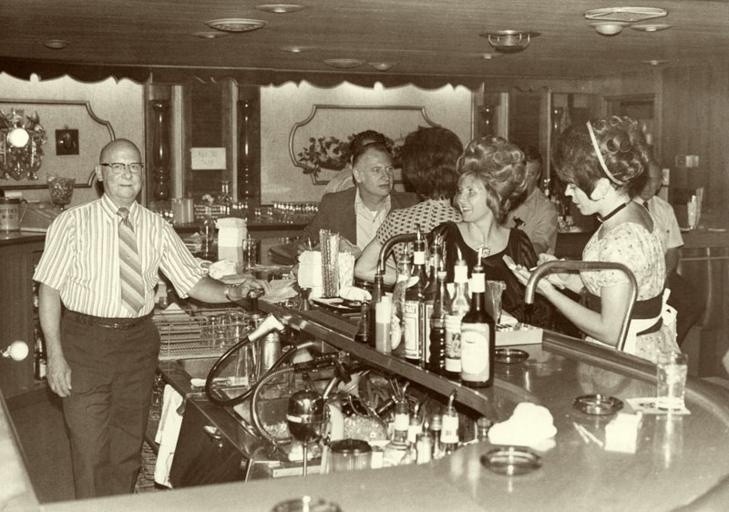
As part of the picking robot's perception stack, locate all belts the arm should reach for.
[63,310,156,331]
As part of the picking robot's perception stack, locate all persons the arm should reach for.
[630,157,685,286]
[291,140,418,256]
[353,124,465,291]
[497,144,556,256]
[423,139,550,327]
[493,113,680,367]
[320,129,397,198]
[33,138,275,502]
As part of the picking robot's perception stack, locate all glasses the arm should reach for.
[99,161,146,175]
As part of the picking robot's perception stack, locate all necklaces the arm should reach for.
[596,198,633,223]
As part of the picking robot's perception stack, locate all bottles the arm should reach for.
[355,222,494,387]
[331,365,490,472]
[33,264,46,383]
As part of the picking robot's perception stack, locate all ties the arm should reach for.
[115,207,148,314]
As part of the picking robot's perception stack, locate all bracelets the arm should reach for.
[224,284,238,303]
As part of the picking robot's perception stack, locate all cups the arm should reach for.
[242,239,256,273]
[656,353,688,411]
[207,312,256,385]
[273,200,320,223]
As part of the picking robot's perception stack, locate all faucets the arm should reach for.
[379,232,426,273]
[523,259,639,350]
